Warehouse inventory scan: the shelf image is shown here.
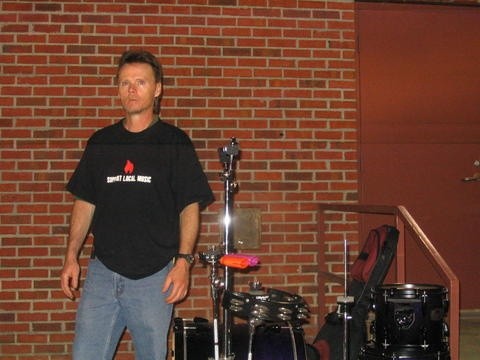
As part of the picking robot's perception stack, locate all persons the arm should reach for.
[59,48,215,359]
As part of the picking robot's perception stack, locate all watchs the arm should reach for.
[176,252,195,265]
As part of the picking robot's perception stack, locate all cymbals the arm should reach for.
[223,281,310,327]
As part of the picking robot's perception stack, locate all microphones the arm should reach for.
[227,135,240,172]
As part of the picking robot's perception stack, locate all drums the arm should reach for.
[359,282,449,360]
